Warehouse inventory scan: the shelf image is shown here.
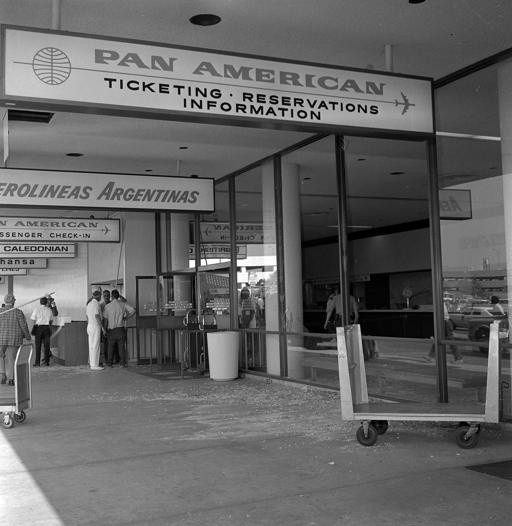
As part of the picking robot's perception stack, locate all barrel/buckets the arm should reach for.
[207,331,241,383]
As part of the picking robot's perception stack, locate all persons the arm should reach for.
[29,297,53,367]
[0,293,32,385]
[238,282,266,370]
[424,299,464,366]
[41,294,59,362]
[490,296,503,316]
[85,289,137,371]
[322,284,359,349]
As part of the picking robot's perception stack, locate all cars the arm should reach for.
[448,306,493,330]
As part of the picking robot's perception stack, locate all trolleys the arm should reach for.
[0,343,34,428]
[336,319,502,448]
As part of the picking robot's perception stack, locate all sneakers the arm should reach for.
[451,359,463,364]
[33,362,51,366]
[0,378,14,386]
[423,355,435,362]
[90,360,126,370]
